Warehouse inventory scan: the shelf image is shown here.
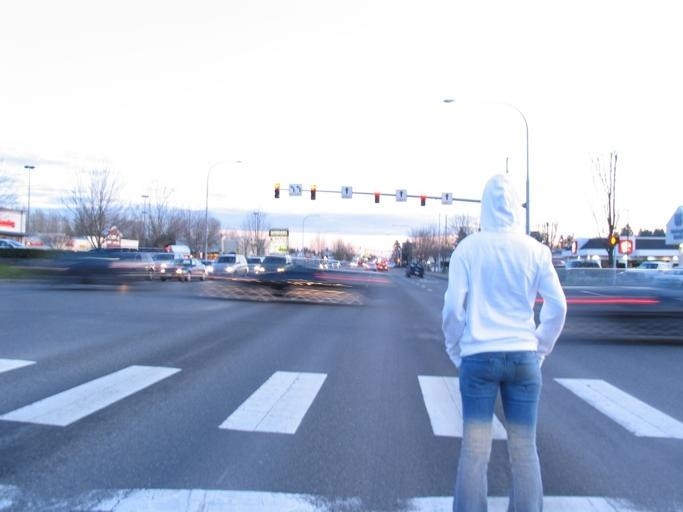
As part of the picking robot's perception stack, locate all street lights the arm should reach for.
[443,98,530,235]
[204,159,243,261]
[141,194,149,229]
[24,164,35,247]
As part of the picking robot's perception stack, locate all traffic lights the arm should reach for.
[610,235,617,246]
[420,194,426,206]
[310,184,316,200]
[374,191,380,203]
[274,183,281,199]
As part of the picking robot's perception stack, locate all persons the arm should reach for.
[443,175,568,512]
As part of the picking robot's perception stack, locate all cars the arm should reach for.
[85,250,294,281]
[623,261,683,290]
[405,263,425,278]
[565,259,605,283]
[365,262,389,272]
